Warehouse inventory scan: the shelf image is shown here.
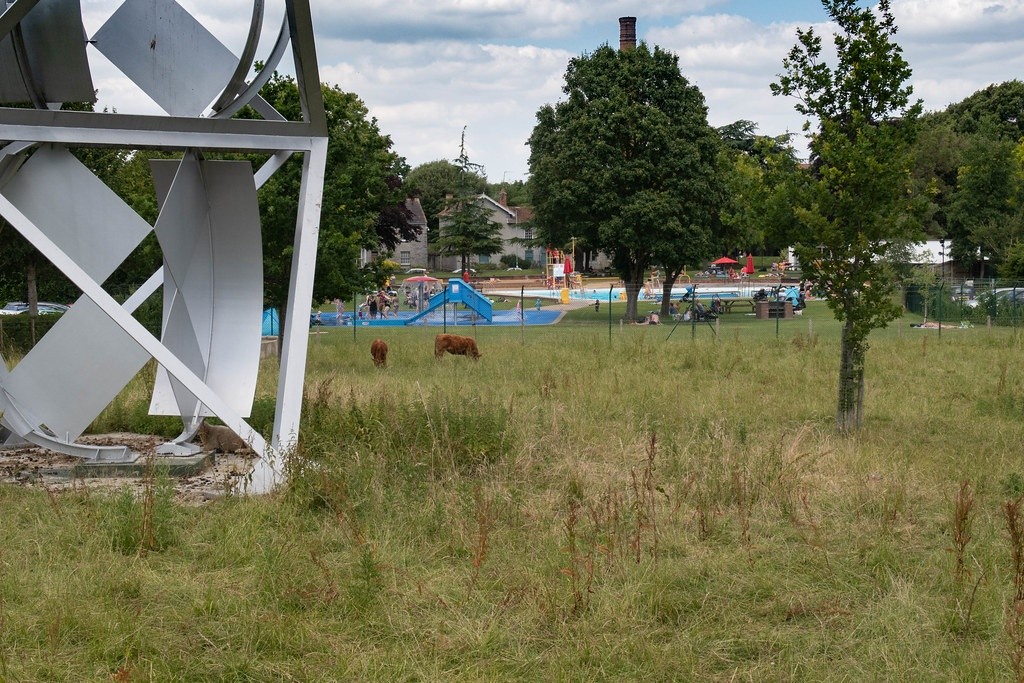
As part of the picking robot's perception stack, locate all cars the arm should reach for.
[962,287,1024,309]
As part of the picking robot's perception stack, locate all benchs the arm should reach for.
[721,305,756,313]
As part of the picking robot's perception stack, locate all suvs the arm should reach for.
[0,302,72,314]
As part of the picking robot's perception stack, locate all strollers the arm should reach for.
[696,294,721,321]
[680,284,699,303]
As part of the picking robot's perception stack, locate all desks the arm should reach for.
[718,296,756,313]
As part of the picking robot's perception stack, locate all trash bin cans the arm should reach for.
[561,288,570,304]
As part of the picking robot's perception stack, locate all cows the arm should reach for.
[371,340,388,369]
[434,334,482,363]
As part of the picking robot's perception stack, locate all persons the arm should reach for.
[335,270,513,326]
[516,299,521,319]
[632,263,815,326]
[316,311,322,321]
[541,245,565,290]
[594,298,600,312]
[534,299,542,311]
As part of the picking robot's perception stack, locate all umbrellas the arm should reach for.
[713,257,739,275]
[563,255,574,289]
[746,253,755,283]
